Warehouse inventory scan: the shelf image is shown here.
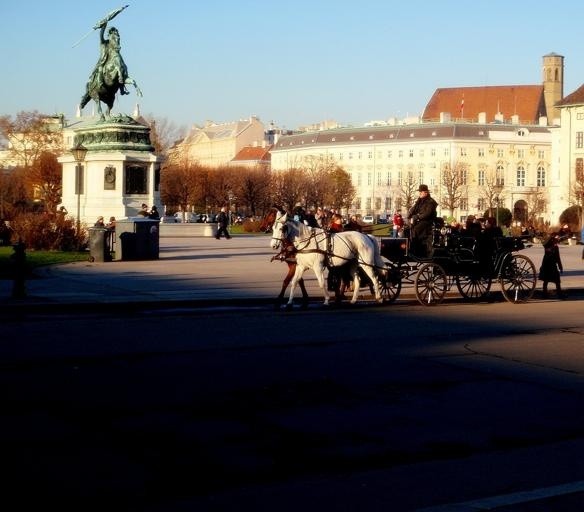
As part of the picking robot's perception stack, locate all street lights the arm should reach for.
[70,142,87,233]
[228,191,235,233]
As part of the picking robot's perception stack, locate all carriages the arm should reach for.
[271,209,536,309]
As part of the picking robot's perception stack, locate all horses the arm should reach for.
[269,205,390,311]
[76,27,143,123]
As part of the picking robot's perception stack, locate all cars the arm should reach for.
[378,218,387,224]
[175,212,214,223]
[363,216,373,223]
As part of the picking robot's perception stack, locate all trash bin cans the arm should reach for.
[85,225,115,263]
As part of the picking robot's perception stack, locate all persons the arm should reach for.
[215,207,232,239]
[94,216,117,231]
[406,185,437,253]
[447,215,503,244]
[137,204,159,219]
[199,212,207,223]
[539,232,565,298]
[392,209,404,238]
[96,22,130,95]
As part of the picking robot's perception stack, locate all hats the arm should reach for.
[415,185,430,192]
